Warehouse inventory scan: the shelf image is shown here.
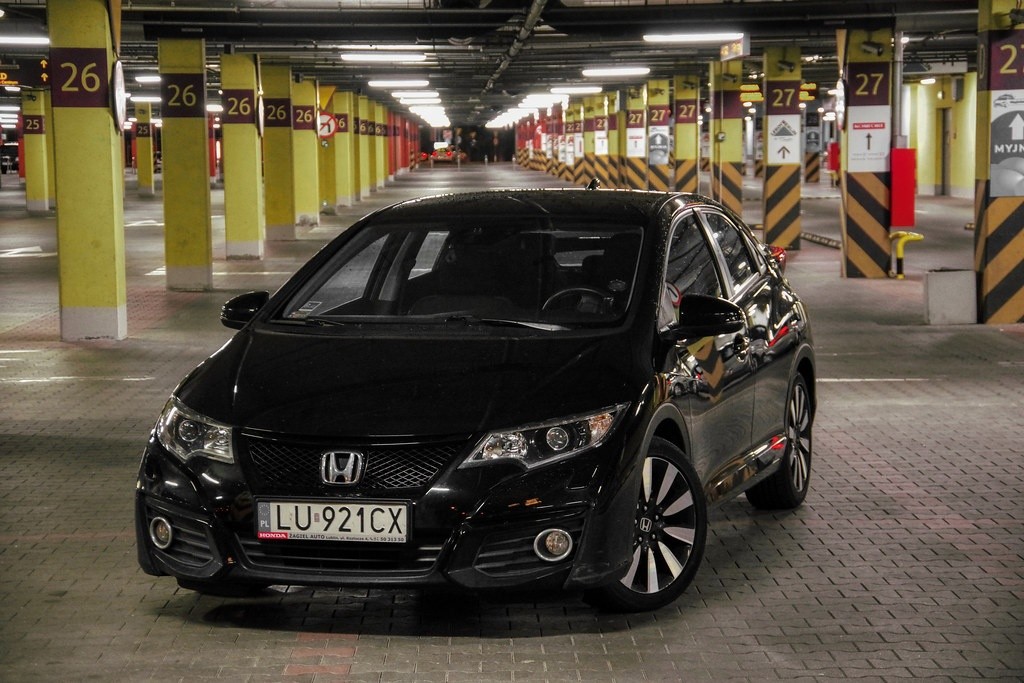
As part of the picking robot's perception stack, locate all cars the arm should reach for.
[420,145,467,162]
[132,176,817,615]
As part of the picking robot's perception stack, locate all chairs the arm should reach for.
[394,227,647,321]
